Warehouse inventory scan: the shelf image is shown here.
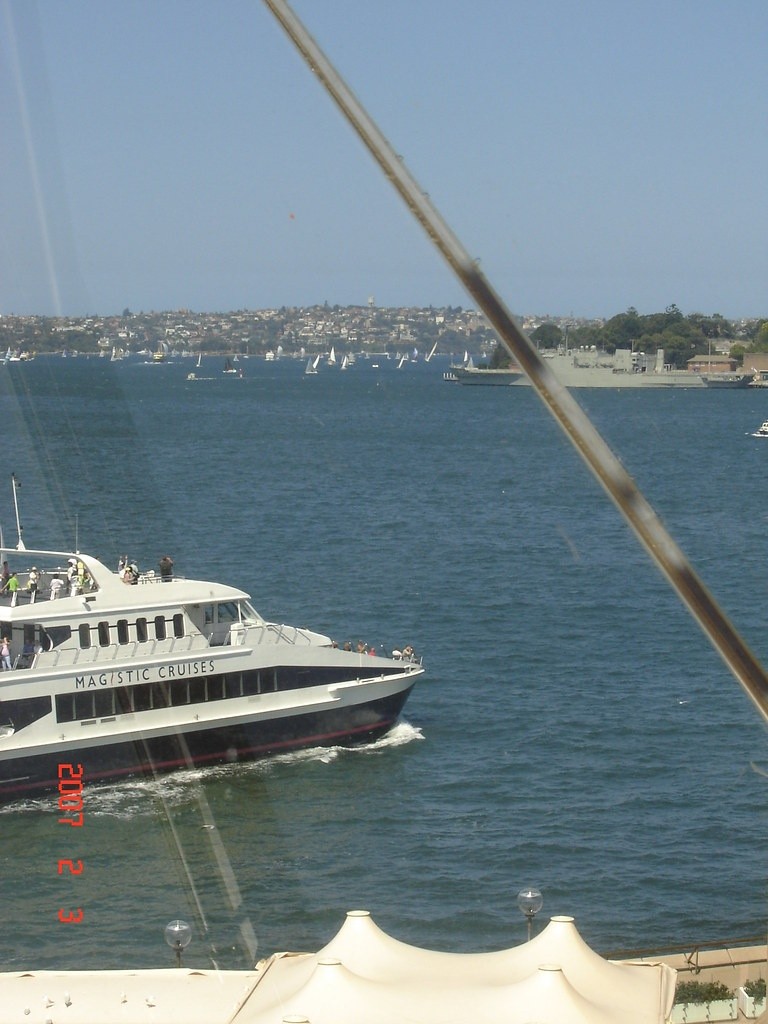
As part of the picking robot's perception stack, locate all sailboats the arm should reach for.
[170,348,189,358]
[425,342,438,362]
[222,360,237,374]
[394,347,418,369]
[305,354,321,374]
[462,350,470,363]
[61,350,79,358]
[194,353,202,367]
[233,354,239,361]
[327,346,356,370]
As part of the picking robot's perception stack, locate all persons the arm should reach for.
[0,637,11,672]
[124,560,140,585]
[392,649,402,661]
[344,641,353,651]
[23,639,33,653]
[403,645,412,662]
[332,640,338,649]
[0,561,10,598]
[118,556,124,571]
[67,559,96,596]
[358,639,372,653]
[158,557,174,583]
[28,567,42,604]
[368,647,376,656]
[33,640,43,654]
[0,572,23,607]
[49,574,64,600]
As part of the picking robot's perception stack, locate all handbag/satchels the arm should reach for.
[31,583,37,592]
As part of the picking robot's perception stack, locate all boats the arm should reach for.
[0,471,425,801]
[110,346,124,362]
[187,374,197,381]
[758,419,768,435]
[449,325,757,388]
[19,351,33,362]
[153,345,167,362]
[98,350,105,357]
[265,350,279,361]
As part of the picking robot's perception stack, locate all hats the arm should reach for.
[126,567,130,570]
[406,644,413,647]
[32,567,37,571]
